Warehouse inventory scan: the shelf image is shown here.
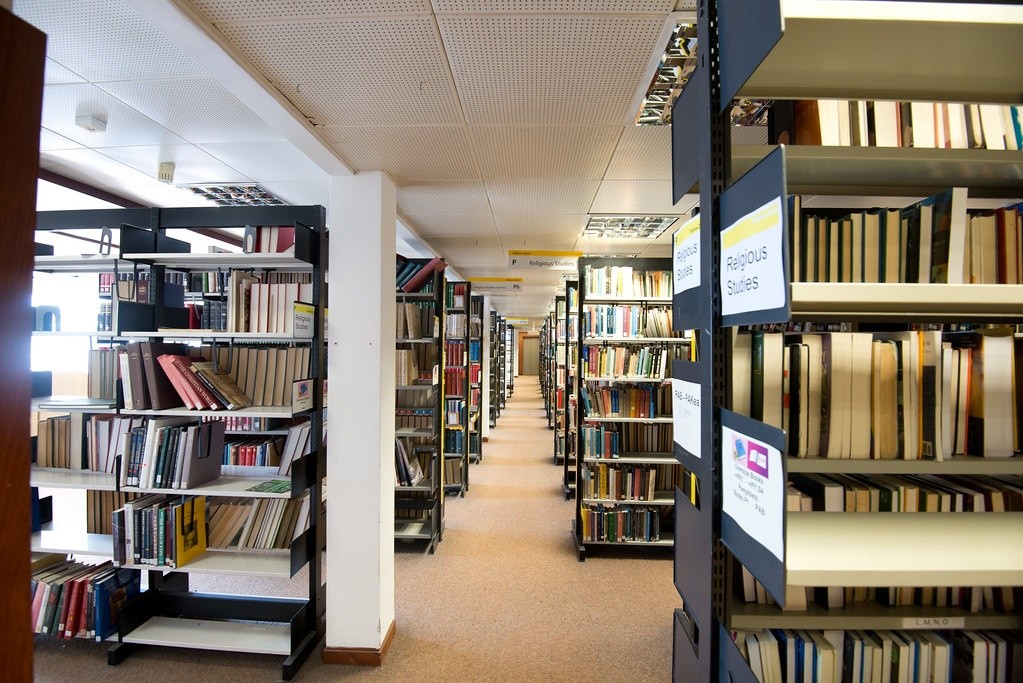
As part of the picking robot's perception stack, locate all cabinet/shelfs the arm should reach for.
[1,0,514,683]
[538,0,1023,682]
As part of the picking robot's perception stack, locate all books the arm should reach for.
[539,264,692,543]
[725,95,1023,683]
[29,262,327,643]
[395,256,504,537]
